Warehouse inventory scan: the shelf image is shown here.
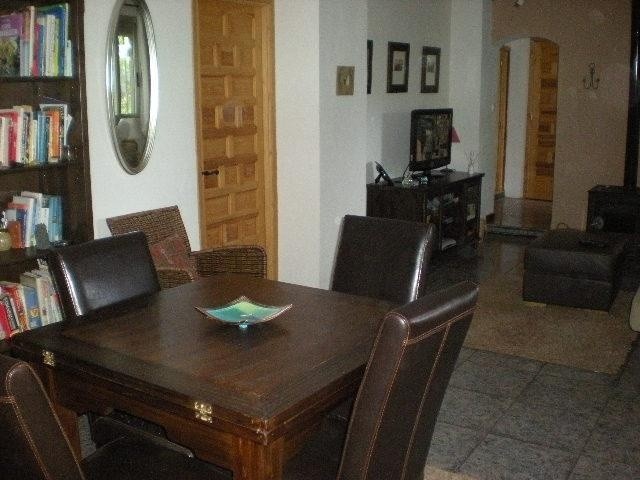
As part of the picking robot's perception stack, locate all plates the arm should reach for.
[194,296,295,327]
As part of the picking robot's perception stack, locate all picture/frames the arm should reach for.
[335,64,355,96]
[366,39,374,95]
[384,39,411,94]
[419,44,442,93]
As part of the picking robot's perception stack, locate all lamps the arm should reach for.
[580,61,602,90]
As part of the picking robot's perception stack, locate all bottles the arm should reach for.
[61,144,78,166]
[1,229,13,253]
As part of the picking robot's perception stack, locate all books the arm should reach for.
[0,1,75,342]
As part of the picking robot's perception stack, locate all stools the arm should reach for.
[517,224,632,314]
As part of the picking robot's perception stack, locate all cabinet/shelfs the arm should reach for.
[0,0,95,367]
[519,37,561,204]
[364,166,485,267]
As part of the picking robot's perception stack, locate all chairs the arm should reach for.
[106,202,270,298]
[284,277,482,479]
[1,354,216,480]
[42,229,170,454]
[299,214,442,477]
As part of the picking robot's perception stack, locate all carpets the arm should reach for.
[416,257,640,380]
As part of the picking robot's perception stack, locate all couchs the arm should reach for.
[582,183,639,294]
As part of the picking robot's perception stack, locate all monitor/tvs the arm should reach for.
[409,108,454,178]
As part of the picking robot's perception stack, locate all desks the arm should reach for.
[0,270,400,478]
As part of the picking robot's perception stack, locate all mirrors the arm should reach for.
[104,0,159,176]
